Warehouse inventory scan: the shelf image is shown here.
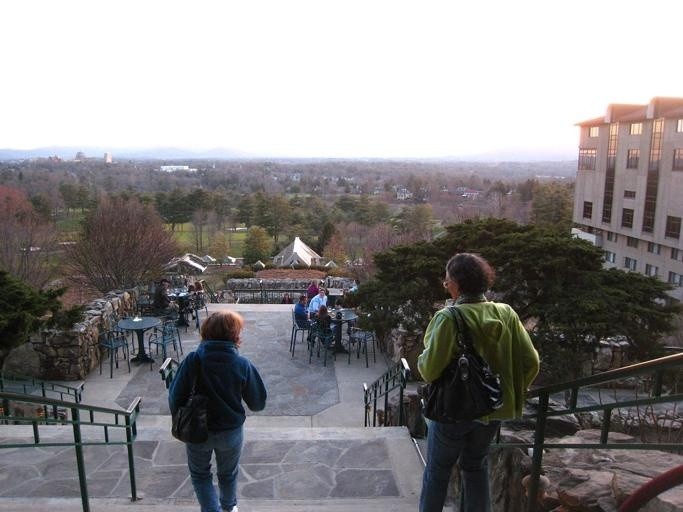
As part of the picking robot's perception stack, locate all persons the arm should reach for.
[167,309,268,511]
[153,279,205,318]
[294,278,345,348]
[417,251,540,511]
[282,293,293,304]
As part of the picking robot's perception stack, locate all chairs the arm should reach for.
[97,279,376,377]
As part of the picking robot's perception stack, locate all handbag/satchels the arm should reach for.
[171,402,209,444]
[417,306,505,424]
